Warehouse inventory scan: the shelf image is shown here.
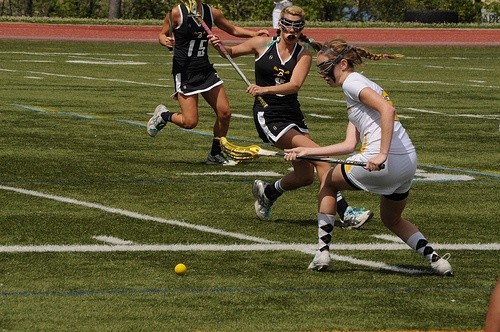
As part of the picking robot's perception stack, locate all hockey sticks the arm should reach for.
[180,0,268,109]
[162,0,176,51]
[220,137,385,169]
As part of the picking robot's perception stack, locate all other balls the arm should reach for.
[174,264,186,275]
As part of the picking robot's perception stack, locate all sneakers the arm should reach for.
[205,150,238,166]
[430,253,454,277]
[339,205,374,230]
[252,179,276,220]
[147,104,170,137]
[307,252,332,271]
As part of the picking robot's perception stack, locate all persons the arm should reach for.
[147,2,270,170]
[307,38,453,276]
[207,5,374,229]
[270,1,294,39]
